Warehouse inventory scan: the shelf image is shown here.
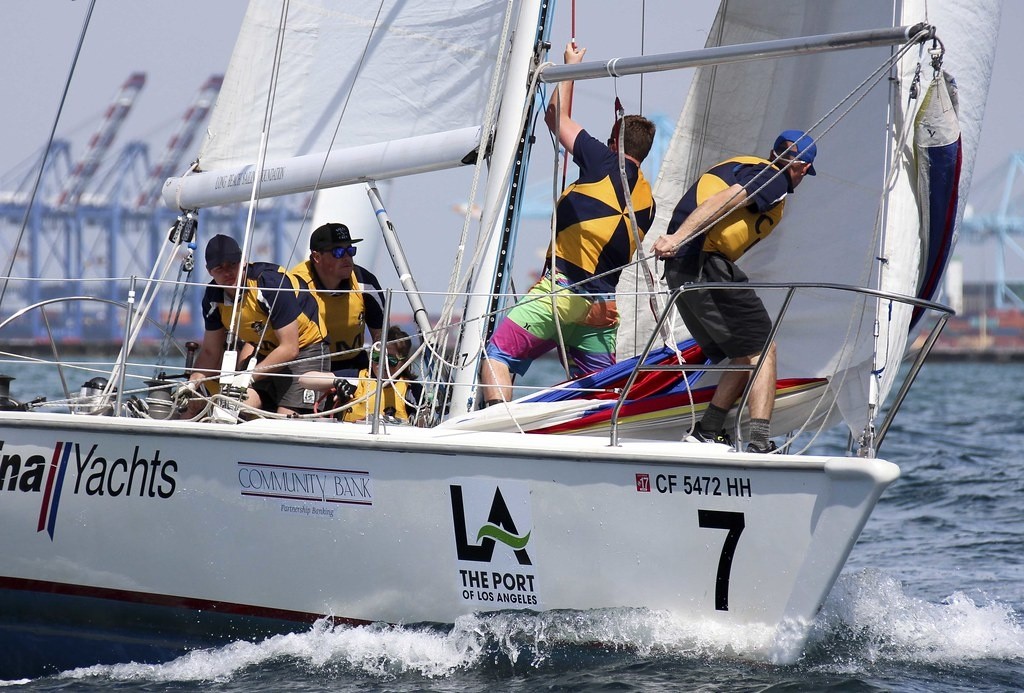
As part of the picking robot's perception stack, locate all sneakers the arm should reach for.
[747,442,782,455]
[681,422,735,449]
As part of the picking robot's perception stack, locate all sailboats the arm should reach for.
[1,1,1000,659]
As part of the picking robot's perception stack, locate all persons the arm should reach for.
[482,45,655,411]
[321,326,433,429]
[174,234,331,419]
[290,223,390,372]
[650,130,816,455]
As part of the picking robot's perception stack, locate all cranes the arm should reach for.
[0,68,317,356]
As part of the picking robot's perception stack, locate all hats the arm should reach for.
[310,224,363,248]
[205,234,241,268]
[774,129,816,176]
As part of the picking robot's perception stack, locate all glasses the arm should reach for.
[371,351,406,367]
[317,246,357,259]
